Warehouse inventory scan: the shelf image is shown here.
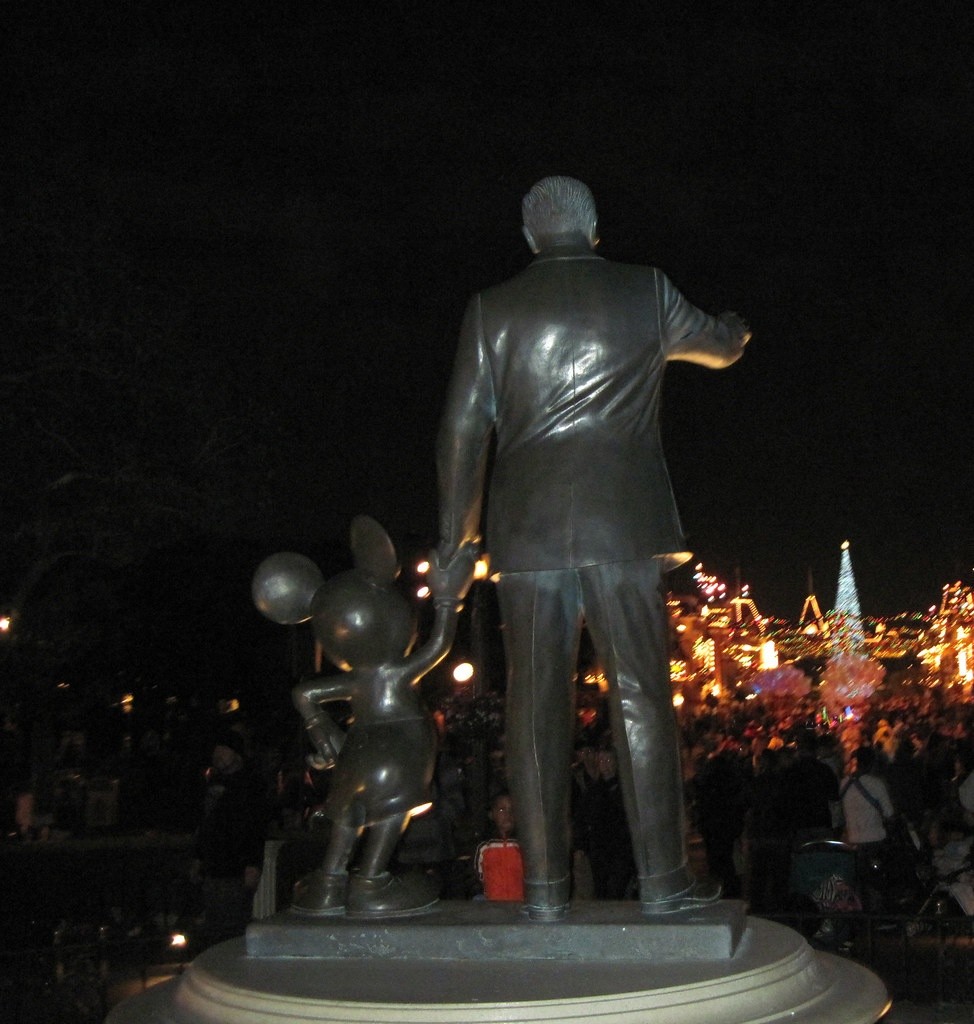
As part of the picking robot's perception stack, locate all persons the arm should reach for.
[431,174,750,922]
[1,627,974,952]
